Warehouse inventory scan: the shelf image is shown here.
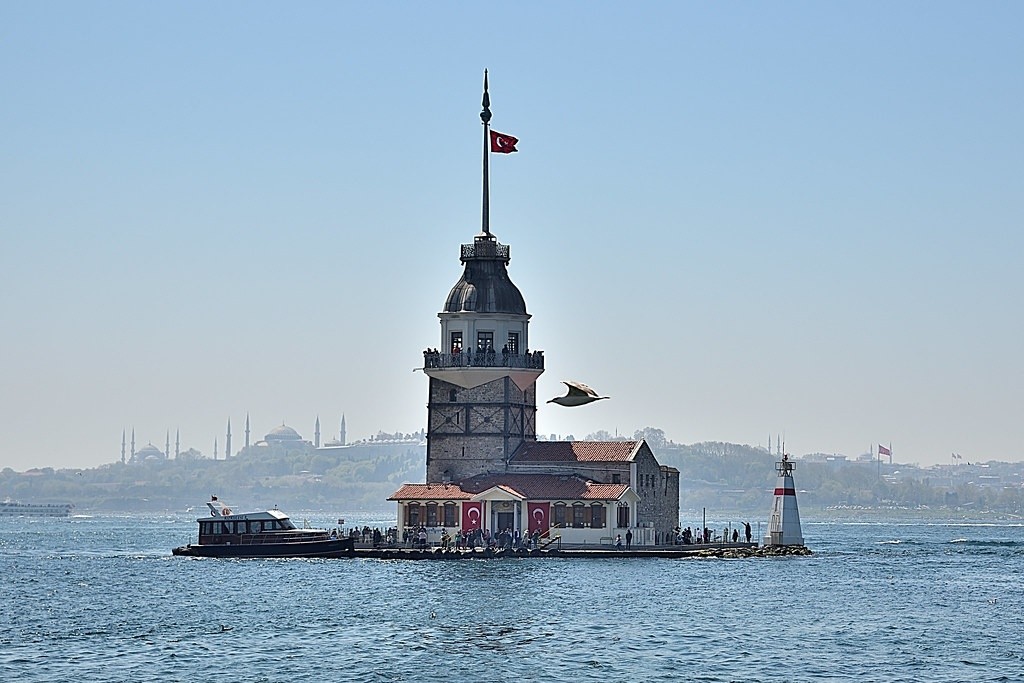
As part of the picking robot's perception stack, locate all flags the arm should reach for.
[878,444,890,456]
[952,453,961,459]
[490,129,520,154]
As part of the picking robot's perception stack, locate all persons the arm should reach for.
[625,529,633,550]
[423,344,510,368]
[746,522,751,543]
[724,528,728,542]
[524,349,544,368]
[732,529,739,542]
[330,525,540,550]
[615,533,623,550]
[673,526,713,544]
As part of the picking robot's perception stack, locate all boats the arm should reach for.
[0,501,75,520]
[171,494,356,561]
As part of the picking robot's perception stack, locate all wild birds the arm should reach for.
[546,381,611,408]
